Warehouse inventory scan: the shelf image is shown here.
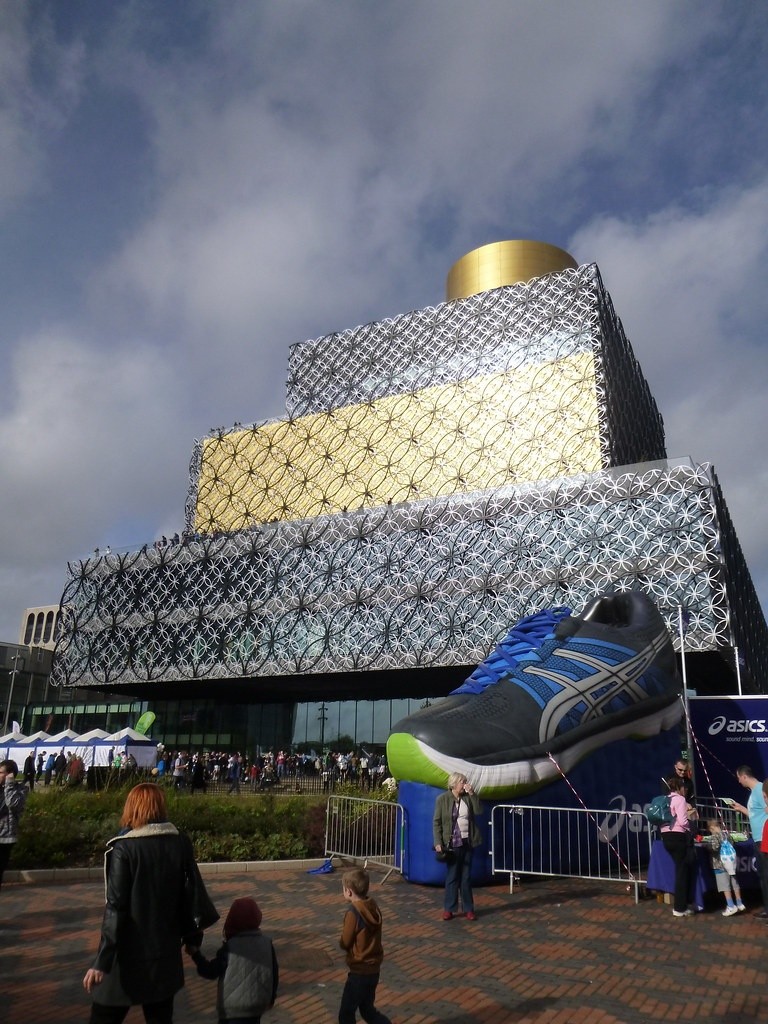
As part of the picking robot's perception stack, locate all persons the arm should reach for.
[185,895,279,1024]
[729,764,768,922]
[153,748,392,796]
[111,754,122,767]
[108,747,115,767]
[0,759,27,888]
[667,758,698,837]
[659,780,699,917]
[707,818,746,917]
[45,752,57,788]
[64,751,85,789]
[433,772,485,921]
[35,751,46,786]
[119,751,128,769]
[127,753,138,778]
[55,751,67,787]
[84,783,221,1023]
[337,869,393,1024]
[23,751,36,793]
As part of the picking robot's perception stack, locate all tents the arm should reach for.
[0,727,159,771]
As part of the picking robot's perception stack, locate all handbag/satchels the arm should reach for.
[435,846,449,863]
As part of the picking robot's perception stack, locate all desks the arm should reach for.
[646,837,757,911]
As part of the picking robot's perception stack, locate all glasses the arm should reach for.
[676,766,688,772]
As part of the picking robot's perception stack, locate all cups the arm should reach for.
[696,833,703,842]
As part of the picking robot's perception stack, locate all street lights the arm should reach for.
[660,604,698,806]
[2,650,23,739]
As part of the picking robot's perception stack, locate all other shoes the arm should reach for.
[466,912,475,921]
[752,910,768,920]
[442,912,453,920]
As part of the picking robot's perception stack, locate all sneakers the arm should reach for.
[385,593,688,805]
[721,904,738,916]
[672,907,695,917]
[737,903,746,913]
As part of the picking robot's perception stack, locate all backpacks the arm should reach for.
[170,757,182,772]
[715,833,737,876]
[644,795,677,830]
